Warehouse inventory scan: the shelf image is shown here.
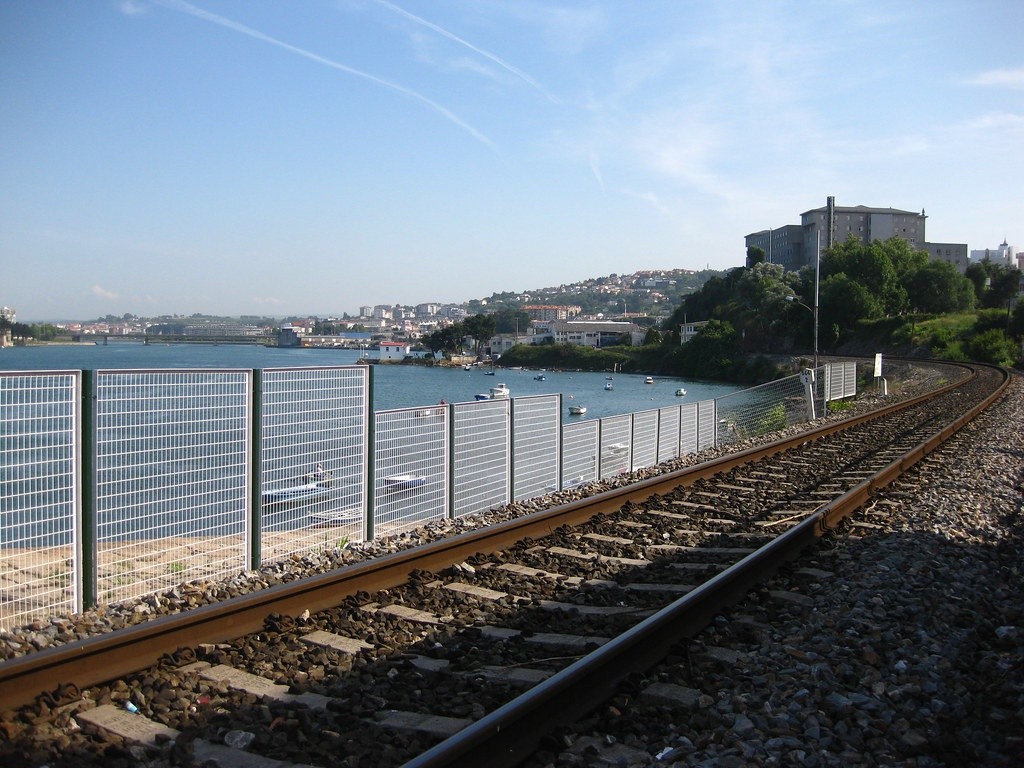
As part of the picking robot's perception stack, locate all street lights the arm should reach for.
[785,296,819,416]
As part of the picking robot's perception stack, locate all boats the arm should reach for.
[645,377,654,384]
[604,383,613,391]
[537,374,545,381]
[308,504,378,528]
[263,469,335,504]
[473,383,511,401]
[675,388,687,396]
[569,406,586,415]
[384,474,427,489]
